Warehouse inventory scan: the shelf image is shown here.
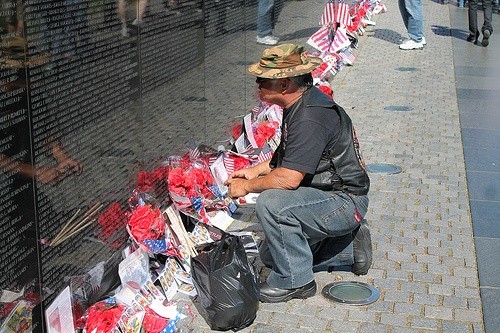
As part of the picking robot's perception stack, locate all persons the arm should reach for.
[228,42,373,304]
[467,0,493,48]
[396,0,426,49]
[440,0,465,8]
[254,0,281,46]
[114,0,147,38]
[2,0,26,38]
[0,35,83,253]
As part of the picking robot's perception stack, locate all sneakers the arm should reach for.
[352,219,372,274]
[405,36,426,44]
[399,40,423,50]
[257,35,278,46]
[259,280,316,304]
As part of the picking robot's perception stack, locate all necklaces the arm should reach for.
[283,93,302,109]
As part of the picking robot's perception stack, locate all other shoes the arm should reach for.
[122,28,133,36]
[482,29,490,47]
[132,20,145,29]
[467,37,477,44]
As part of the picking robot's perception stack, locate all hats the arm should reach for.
[248,44,322,80]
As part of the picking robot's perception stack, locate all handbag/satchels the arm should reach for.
[190,234,259,331]
